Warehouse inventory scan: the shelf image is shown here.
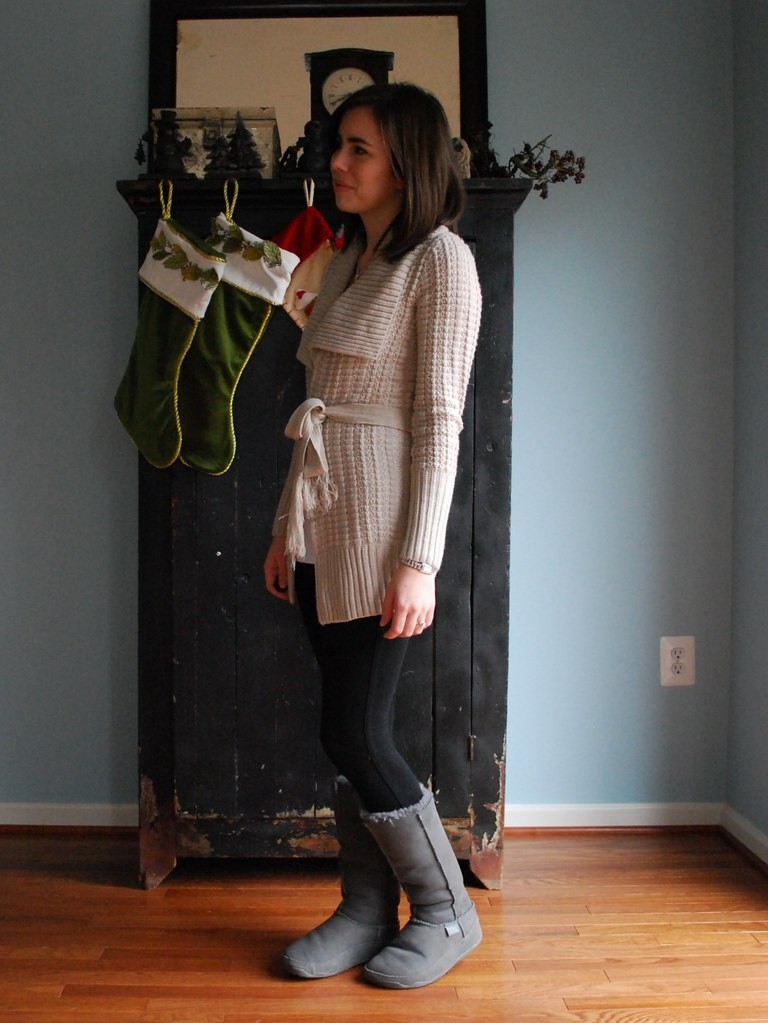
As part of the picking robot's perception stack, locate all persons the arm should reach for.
[263,82,480,990]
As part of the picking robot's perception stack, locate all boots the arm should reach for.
[281,775,400,979]
[358,782,483,989]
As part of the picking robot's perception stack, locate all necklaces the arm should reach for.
[355,254,363,278]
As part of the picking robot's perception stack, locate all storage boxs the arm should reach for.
[150,107,283,179]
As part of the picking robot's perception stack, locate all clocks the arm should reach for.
[304,48,394,173]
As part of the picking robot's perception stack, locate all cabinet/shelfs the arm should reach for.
[112,178,534,891]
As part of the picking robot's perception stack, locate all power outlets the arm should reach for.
[658,635,695,688]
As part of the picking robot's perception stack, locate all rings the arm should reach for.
[417,622,426,626]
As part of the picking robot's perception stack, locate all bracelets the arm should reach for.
[402,559,433,575]
